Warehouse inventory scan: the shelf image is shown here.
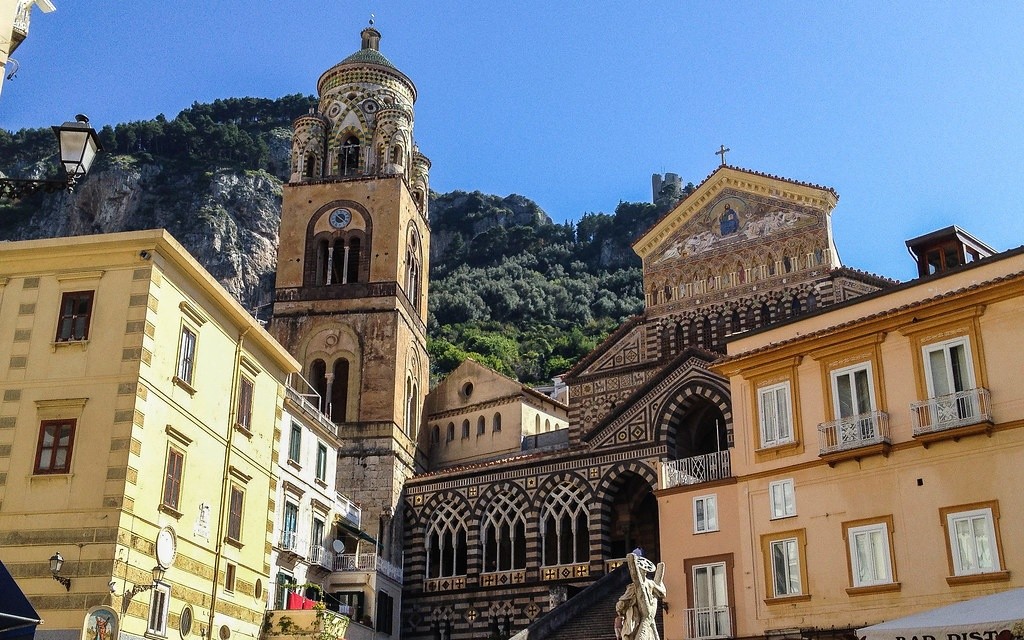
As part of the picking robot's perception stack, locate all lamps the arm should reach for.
[48,551,71,592]
[0,113,106,229]
[125,564,167,599]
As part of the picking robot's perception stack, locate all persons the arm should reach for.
[616,553,667,640]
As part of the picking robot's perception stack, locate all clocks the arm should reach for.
[329,208,352,228]
